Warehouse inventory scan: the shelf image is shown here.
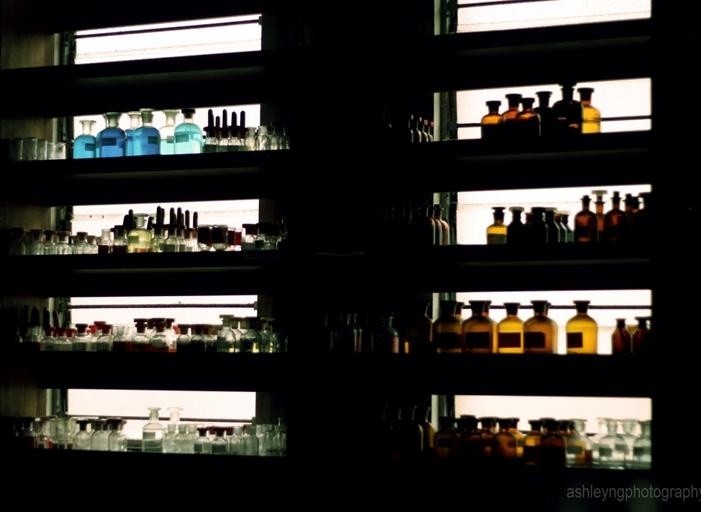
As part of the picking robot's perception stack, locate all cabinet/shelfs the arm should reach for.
[2,19,700,507]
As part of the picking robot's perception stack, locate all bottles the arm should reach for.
[428,411,652,470]
[14,408,288,462]
[7,107,291,161]
[486,193,656,251]
[401,112,439,143]
[423,292,654,360]
[480,85,608,144]
[8,205,289,253]
[8,299,288,356]
[366,402,425,461]
[391,196,457,246]
[322,310,411,357]
